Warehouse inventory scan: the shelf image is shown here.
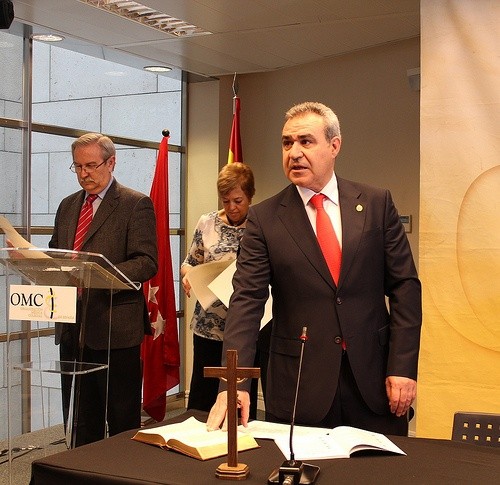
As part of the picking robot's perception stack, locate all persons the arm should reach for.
[180,162,260,420]
[44,133,158,451]
[205,103,423,437]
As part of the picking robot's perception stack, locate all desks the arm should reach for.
[29,409,500,485]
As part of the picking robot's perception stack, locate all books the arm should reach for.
[272,422,410,461]
[131,415,260,459]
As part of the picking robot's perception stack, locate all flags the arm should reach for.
[216,72,255,164]
[141,129,177,422]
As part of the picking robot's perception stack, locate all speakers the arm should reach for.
[0,0,14,29]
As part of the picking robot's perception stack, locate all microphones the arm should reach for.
[267,327,320,485]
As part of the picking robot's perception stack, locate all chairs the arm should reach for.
[452,412,500,448]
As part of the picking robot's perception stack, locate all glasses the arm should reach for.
[69,154,113,173]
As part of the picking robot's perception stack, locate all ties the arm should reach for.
[72,194,98,299]
[309,194,347,350]
